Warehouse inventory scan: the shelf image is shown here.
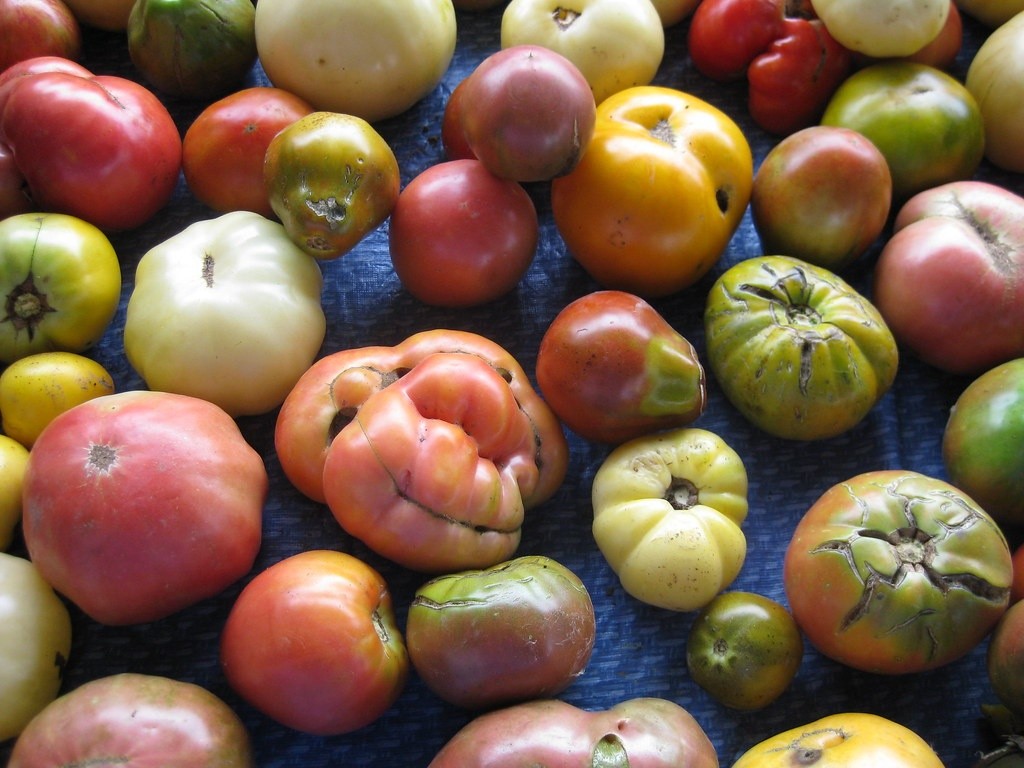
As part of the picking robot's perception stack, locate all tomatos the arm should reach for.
[0,0,1024,768]
